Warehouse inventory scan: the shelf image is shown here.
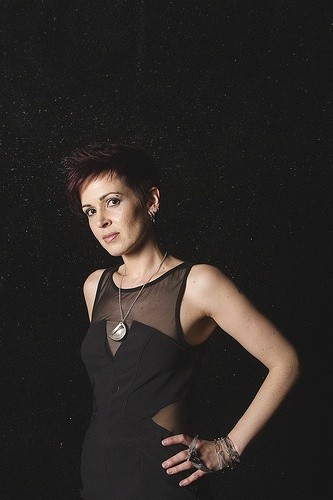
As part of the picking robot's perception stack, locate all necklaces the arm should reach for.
[110,250,170,342]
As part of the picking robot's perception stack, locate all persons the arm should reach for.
[81,144,299,500]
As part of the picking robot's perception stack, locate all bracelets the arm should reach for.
[187,435,240,473]
